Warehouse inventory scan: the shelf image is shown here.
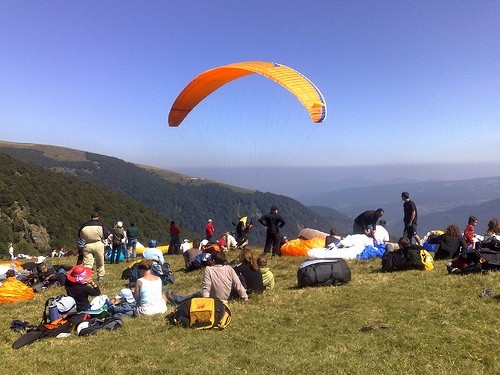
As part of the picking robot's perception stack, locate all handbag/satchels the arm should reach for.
[296,258,351,289]
[43,295,78,324]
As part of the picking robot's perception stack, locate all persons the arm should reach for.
[142,239,165,266]
[77,221,138,265]
[256,257,276,288]
[428,224,468,260]
[353,208,384,235]
[229,249,265,293]
[168,215,253,272]
[463,216,478,246]
[401,191,422,247]
[166,251,250,304]
[258,207,288,255]
[132,258,169,317]
[383,235,424,256]
[326,229,340,248]
[367,220,390,246]
[486,219,500,236]
[79,210,109,281]
[65,264,100,309]
[0,243,74,290]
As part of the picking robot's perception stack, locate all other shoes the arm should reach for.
[98,278,105,282]
[126,260,130,263]
[109,261,119,264]
[165,291,177,304]
[133,259,136,262]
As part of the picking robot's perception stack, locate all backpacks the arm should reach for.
[407,246,434,271]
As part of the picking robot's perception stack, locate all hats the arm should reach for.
[117,221,123,227]
[184,238,190,242]
[37,263,46,272]
[66,265,92,282]
[149,240,157,248]
